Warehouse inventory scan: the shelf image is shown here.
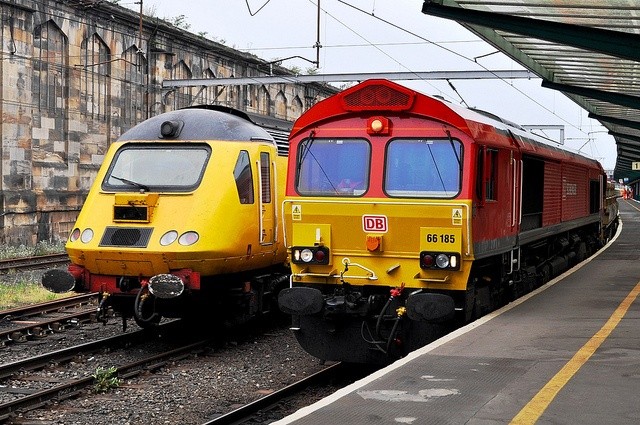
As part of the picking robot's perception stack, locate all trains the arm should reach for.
[43,105,295,334]
[278,78,622,365]
